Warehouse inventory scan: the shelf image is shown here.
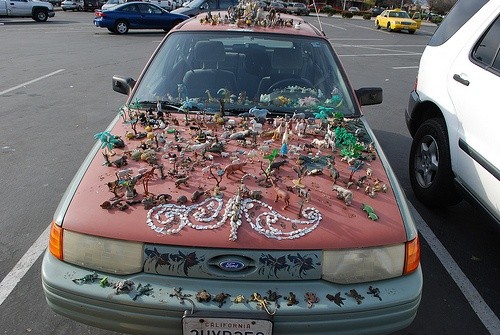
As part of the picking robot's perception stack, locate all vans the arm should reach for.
[286,2,310,16]
[307,3,326,10]
[266,2,286,12]
[101,0,127,10]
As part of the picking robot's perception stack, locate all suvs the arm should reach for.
[168,0,239,17]
[405,0,500,227]
[0,0,55,22]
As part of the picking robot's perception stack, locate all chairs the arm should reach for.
[257,49,314,96]
[182,40,239,99]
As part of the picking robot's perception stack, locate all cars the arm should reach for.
[409,10,446,19]
[370,7,384,15]
[93,2,190,34]
[374,9,422,35]
[60,0,84,12]
[349,7,360,12]
[83,0,96,12]
[40,10,425,335]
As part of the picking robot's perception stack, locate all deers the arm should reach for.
[270,177,290,209]
